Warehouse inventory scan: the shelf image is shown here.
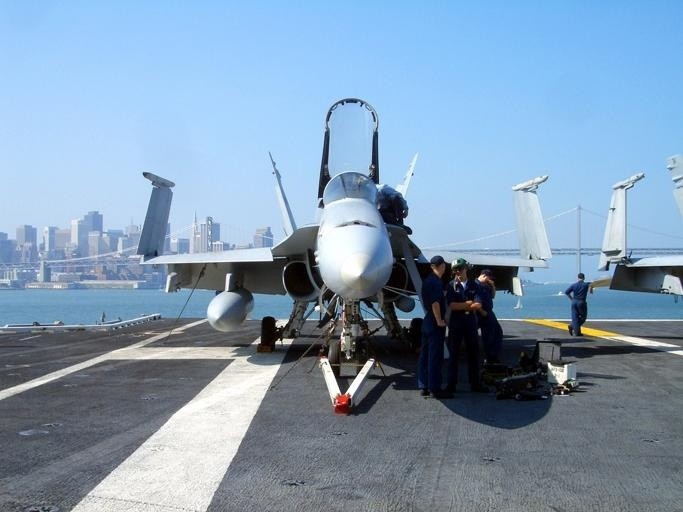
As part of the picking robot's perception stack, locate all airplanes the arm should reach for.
[128,98,551,415]
[596,154,683,303]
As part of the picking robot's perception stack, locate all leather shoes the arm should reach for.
[470,384,489,392]
[569,325,572,336]
[443,385,456,393]
[576,333,583,336]
[419,388,429,396]
[429,390,453,398]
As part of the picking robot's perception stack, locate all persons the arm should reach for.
[417,255,454,399]
[444,258,489,394]
[564,273,592,336]
[473,269,503,367]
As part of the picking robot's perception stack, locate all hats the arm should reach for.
[451,257,466,269]
[430,256,447,265]
[577,273,584,279]
[480,269,497,280]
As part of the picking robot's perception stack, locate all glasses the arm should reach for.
[453,267,465,273]
